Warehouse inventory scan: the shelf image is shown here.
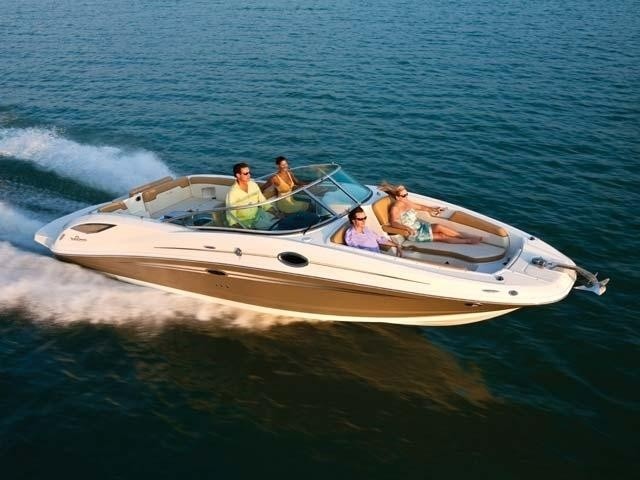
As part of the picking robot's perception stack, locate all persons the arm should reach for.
[261,155,314,214]
[225,163,284,230]
[345,206,402,257]
[377,181,483,243]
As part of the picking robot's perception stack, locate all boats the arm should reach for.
[32,161,611,329]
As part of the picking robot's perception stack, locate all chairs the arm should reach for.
[331,196,509,270]
[97,175,276,227]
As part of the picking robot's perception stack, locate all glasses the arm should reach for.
[399,193,408,197]
[355,217,366,221]
[242,172,251,175]
